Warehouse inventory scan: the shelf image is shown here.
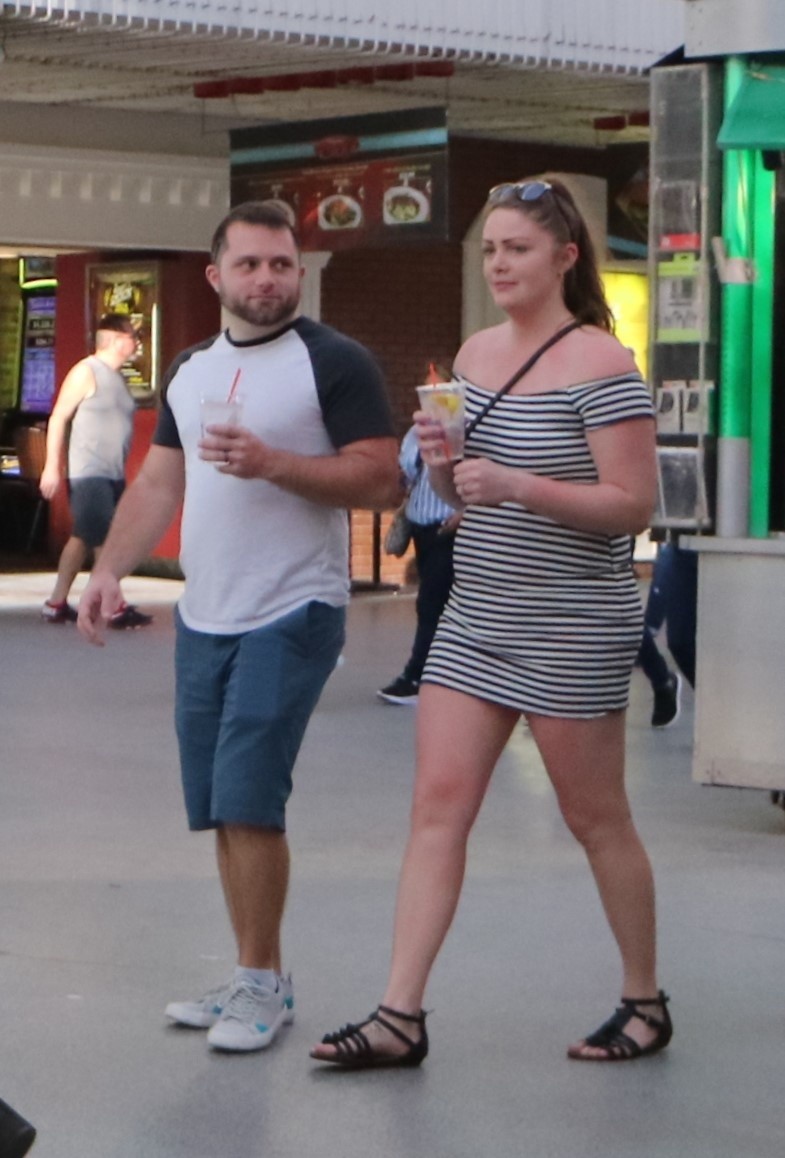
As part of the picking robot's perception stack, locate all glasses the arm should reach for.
[488,180,573,240]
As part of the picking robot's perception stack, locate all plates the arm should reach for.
[316,195,361,230]
[383,186,430,225]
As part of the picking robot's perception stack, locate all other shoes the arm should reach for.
[650,674,681,729]
[41,600,78,625]
[106,604,152,631]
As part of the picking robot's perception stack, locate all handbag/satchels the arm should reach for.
[382,496,411,559]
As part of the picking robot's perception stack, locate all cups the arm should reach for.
[415,380,466,464]
[199,392,245,465]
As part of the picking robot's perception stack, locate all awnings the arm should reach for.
[715,51,785,149]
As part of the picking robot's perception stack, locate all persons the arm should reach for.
[78,199,408,1050]
[376,358,467,704]
[308,180,673,1069]
[635,540,698,728]
[40,315,154,629]
[0,1097,36,1158]
[635,529,698,730]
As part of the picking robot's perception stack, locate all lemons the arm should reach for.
[436,394,459,411]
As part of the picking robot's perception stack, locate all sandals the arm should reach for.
[309,1005,429,1069]
[567,989,670,1061]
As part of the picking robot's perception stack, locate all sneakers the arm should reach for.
[375,675,420,706]
[165,974,294,1052]
